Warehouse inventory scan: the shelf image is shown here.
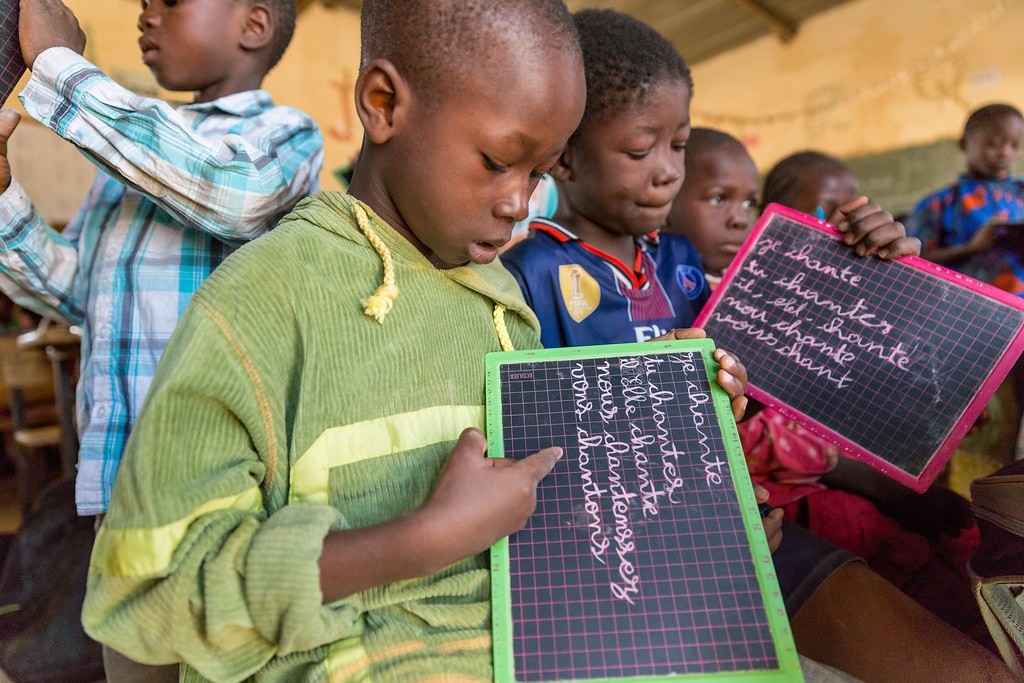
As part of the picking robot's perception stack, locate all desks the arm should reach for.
[15,323,80,484]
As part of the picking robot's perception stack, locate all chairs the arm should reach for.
[0,332,64,521]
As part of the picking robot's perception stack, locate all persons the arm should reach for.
[495,1,1023,682]
[757,146,861,225]
[0,0,322,683]
[659,127,1024,669]
[76,0,753,683]
[898,101,1024,503]
